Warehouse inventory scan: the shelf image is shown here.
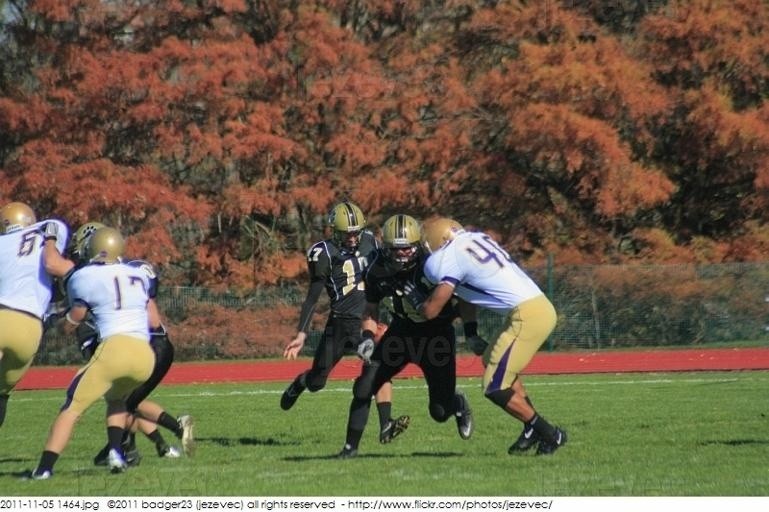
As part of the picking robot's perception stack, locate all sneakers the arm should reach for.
[336,394,566,460]
[281,377,303,410]
[32,416,195,480]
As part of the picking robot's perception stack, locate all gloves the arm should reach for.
[463,321,487,356]
[400,281,424,310]
[356,329,374,365]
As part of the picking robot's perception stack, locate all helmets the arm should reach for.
[2,200,125,264]
[330,202,466,256]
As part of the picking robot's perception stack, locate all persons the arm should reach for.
[42,269,180,466]
[1,201,76,428]
[26,225,160,481]
[281,202,410,445]
[336,214,474,460]
[402,217,567,455]
[69,222,194,466]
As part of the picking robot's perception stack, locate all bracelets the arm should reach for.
[64,313,77,325]
[362,331,375,342]
[464,320,478,338]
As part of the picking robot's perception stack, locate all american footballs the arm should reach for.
[361,323,389,346]
